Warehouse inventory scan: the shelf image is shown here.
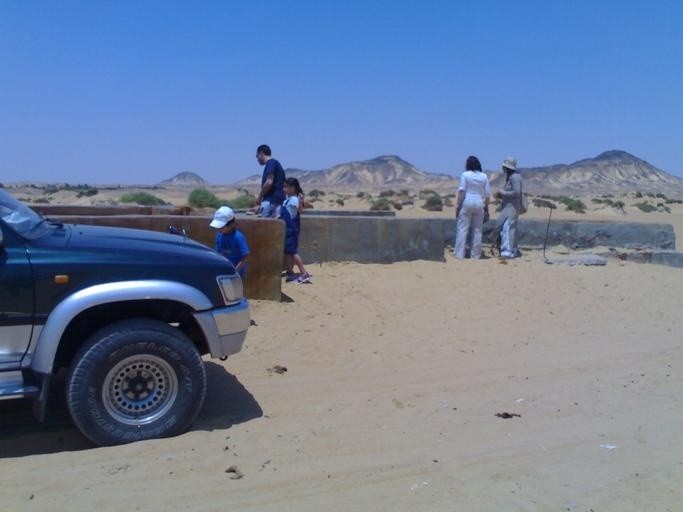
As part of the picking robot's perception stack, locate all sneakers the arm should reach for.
[282,271,312,284]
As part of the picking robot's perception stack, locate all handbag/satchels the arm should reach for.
[519,193,527,214]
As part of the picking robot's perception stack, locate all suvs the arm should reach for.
[0,183,252,449]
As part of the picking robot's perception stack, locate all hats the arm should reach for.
[209,206,234,229]
[502,159,517,171]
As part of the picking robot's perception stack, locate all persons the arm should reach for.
[452,155,524,261]
[244,144,312,285]
[209,206,250,283]
